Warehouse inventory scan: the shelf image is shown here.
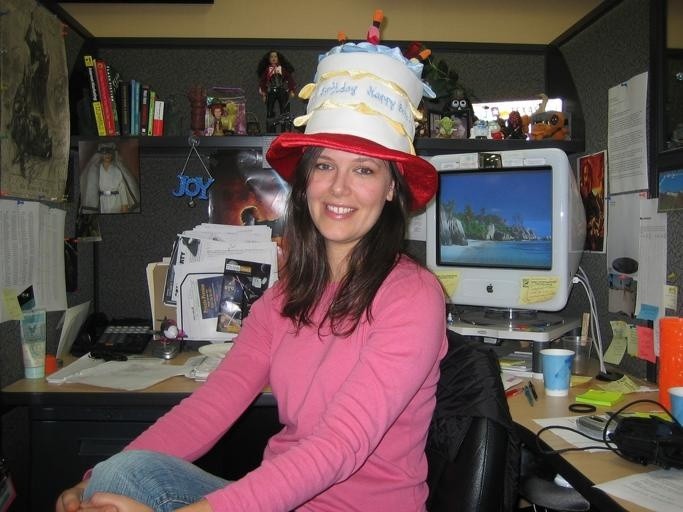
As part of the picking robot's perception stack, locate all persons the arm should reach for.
[579,160,603,250]
[207,98,237,137]
[256,51,296,134]
[53,144,448,511]
[79,142,139,213]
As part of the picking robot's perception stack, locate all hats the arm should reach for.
[266,42,439,212]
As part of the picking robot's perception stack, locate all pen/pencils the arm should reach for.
[528,381,538,401]
[524,384,534,407]
[505,387,524,397]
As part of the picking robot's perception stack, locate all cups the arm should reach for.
[540,347,575,397]
[666,385,683,428]
[561,334,592,376]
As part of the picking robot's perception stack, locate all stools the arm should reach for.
[520,473,589,512]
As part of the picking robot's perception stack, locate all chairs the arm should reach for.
[423,341,521,512]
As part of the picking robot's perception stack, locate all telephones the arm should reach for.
[69,312,153,356]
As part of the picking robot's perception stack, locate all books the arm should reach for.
[499,350,532,390]
[83,54,163,137]
[45,221,278,392]
[657,316,683,410]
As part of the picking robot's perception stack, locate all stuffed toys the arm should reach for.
[435,88,570,142]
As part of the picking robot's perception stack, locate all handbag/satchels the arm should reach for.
[608,416,682,467]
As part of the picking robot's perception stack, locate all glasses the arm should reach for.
[95,147,118,154]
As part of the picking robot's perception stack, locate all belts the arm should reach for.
[98,190,119,194]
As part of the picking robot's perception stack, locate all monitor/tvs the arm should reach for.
[426,148,587,313]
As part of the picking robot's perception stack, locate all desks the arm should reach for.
[0,336,682,512]
